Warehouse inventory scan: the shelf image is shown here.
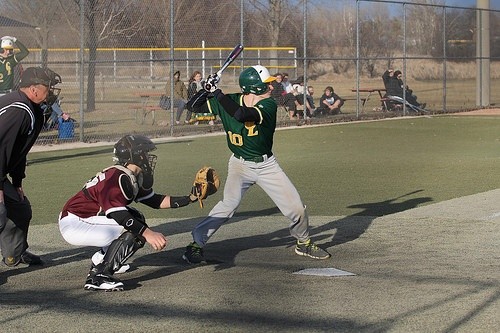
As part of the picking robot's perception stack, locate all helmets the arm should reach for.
[239,66,278,96]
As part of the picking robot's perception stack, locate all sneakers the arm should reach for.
[182,245,206,265]
[295,240,329,259]
[84,273,123,291]
[4,254,42,266]
[90,262,131,273]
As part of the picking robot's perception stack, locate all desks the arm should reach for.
[351,88,390,114]
[136,92,165,125]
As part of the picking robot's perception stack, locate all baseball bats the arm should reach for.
[206,43,245,91]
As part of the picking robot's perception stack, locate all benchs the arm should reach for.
[339,96,391,106]
[127,105,179,126]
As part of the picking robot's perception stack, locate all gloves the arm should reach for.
[205,73,219,93]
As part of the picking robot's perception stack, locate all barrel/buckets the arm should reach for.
[57,120,75,139]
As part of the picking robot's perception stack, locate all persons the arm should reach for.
[394,70,417,100]
[165,69,192,126]
[306,86,321,117]
[41,84,71,131]
[320,86,344,115]
[0,67,62,284]
[289,75,316,118]
[281,72,317,120]
[271,73,304,120]
[58,134,219,293]
[382,69,423,113]
[187,71,214,126]
[183,65,331,266]
[0,35,29,96]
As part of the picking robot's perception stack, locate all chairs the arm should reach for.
[276,105,290,122]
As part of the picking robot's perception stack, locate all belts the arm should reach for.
[60,211,68,219]
[234,152,274,164]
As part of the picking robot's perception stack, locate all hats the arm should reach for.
[0,40,14,51]
[19,67,41,88]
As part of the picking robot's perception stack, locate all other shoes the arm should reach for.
[184,120,190,125]
[296,106,303,110]
[419,103,426,108]
[176,121,181,125]
[310,107,316,115]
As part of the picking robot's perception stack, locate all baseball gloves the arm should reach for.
[194,167,221,200]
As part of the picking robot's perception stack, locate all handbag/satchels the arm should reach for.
[159,95,170,110]
